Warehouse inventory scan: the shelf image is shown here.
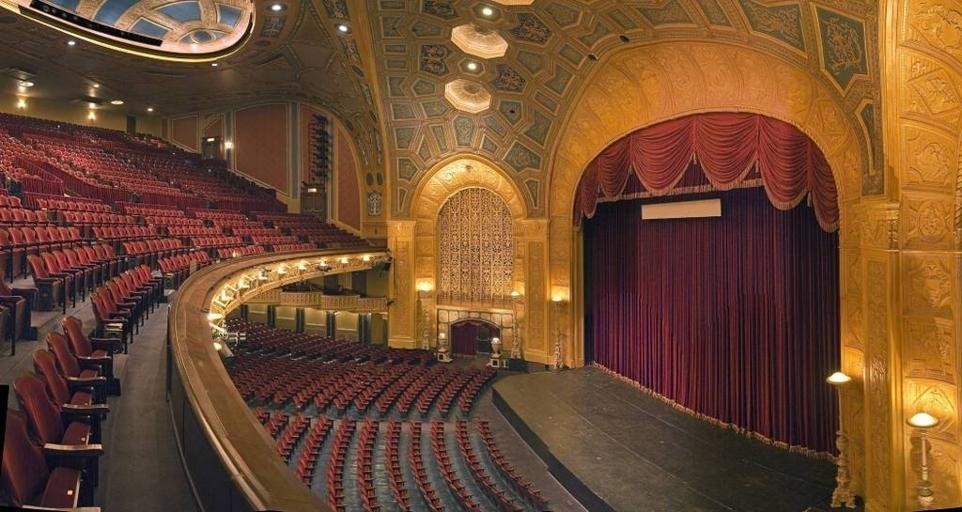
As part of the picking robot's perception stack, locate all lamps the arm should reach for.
[547,296,570,371]
[826,368,858,511]
[905,411,942,511]
[506,290,524,359]
[417,287,435,351]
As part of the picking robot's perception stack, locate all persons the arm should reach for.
[852,495,865,512]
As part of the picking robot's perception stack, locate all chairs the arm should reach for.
[213,308,556,512]
[2,313,127,512]
[2,112,379,355]
